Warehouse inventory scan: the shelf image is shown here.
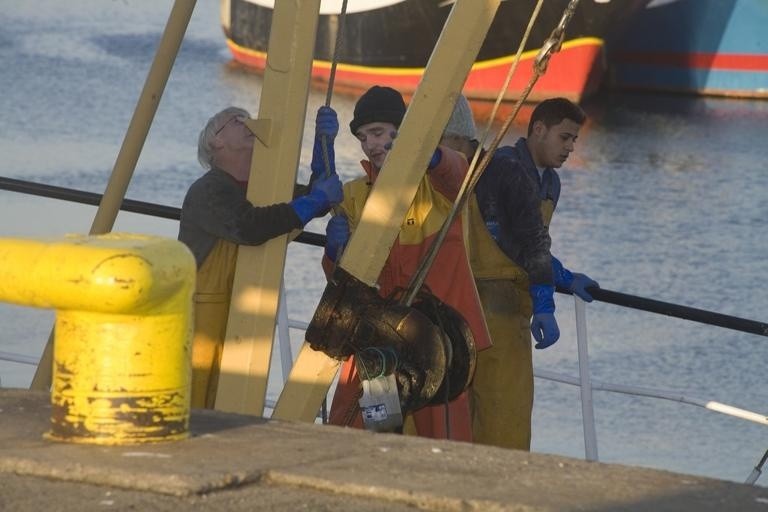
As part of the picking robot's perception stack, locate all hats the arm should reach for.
[443,92,478,140]
[349,85,406,134]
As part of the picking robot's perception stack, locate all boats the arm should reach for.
[221,0,652,110]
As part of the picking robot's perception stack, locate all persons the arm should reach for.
[177,108,344,269]
[438,92,559,453]
[321,84,493,445]
[497,97,600,303]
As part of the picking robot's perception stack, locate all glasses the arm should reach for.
[215,115,251,138]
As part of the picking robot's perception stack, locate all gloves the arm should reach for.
[325,215,350,262]
[550,255,600,304]
[311,106,339,173]
[288,173,345,226]
[384,129,442,169]
[529,284,561,350]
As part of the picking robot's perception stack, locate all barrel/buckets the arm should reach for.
[358,374,403,433]
